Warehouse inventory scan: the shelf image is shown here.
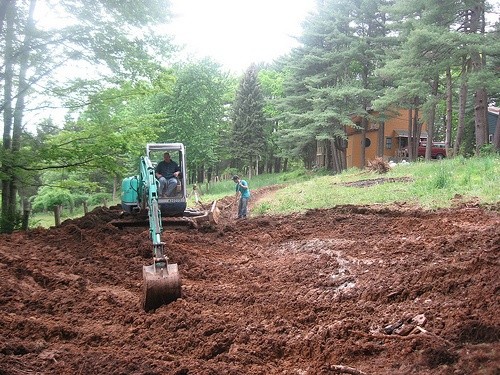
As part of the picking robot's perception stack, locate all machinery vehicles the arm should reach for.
[109,141,198,309]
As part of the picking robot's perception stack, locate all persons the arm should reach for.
[193,187,200,206]
[155,152,181,198]
[232,175,251,219]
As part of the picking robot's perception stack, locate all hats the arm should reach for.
[231,175,239,180]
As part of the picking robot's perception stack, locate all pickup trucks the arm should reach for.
[403,141,446,160]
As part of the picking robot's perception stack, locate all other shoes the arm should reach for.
[158,194,162,199]
[163,193,168,198]
